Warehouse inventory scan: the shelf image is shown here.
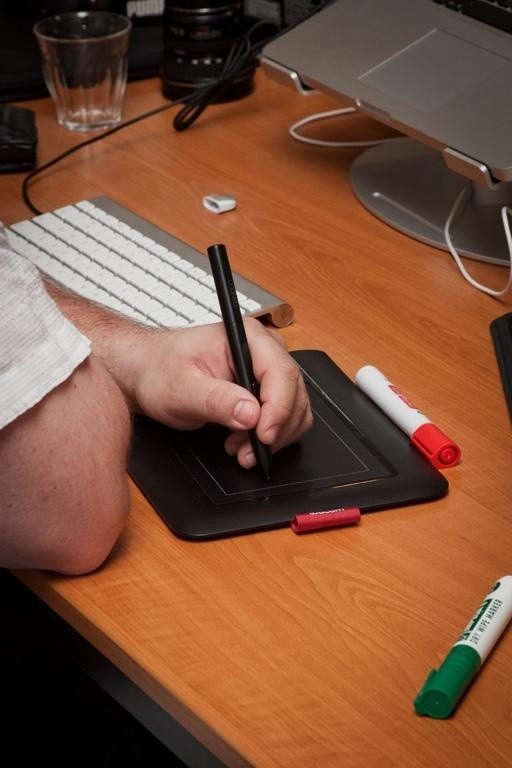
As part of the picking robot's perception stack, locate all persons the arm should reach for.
[1,235,314,577]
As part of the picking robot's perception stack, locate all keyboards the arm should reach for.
[3,193,294,331]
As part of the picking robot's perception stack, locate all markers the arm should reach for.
[412,575,512,719]
[353,364,461,471]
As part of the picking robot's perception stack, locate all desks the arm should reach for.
[0,65,512,766]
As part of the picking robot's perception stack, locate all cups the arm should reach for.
[35,12,133,130]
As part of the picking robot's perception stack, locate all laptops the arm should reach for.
[262,0,512,184]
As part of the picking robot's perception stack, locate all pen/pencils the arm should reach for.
[206,243,271,481]
